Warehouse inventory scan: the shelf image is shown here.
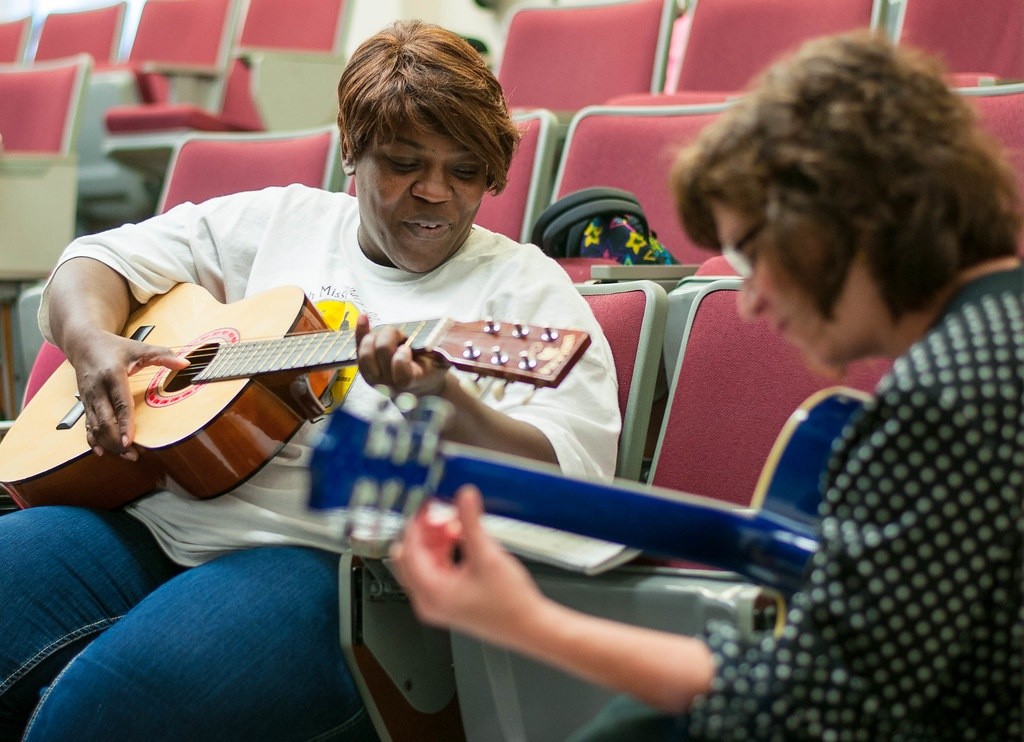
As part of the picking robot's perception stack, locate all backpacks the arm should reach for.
[532,187,681,266]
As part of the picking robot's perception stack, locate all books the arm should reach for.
[479,513,642,574]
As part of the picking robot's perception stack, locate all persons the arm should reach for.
[387,28,1024,742]
[1,16,626,742]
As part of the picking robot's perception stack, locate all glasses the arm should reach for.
[722,218,768,279]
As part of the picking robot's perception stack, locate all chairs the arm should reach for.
[0,0,1024,742]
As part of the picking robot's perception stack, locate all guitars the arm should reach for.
[0,282,591,515]
[303,381,879,639]
